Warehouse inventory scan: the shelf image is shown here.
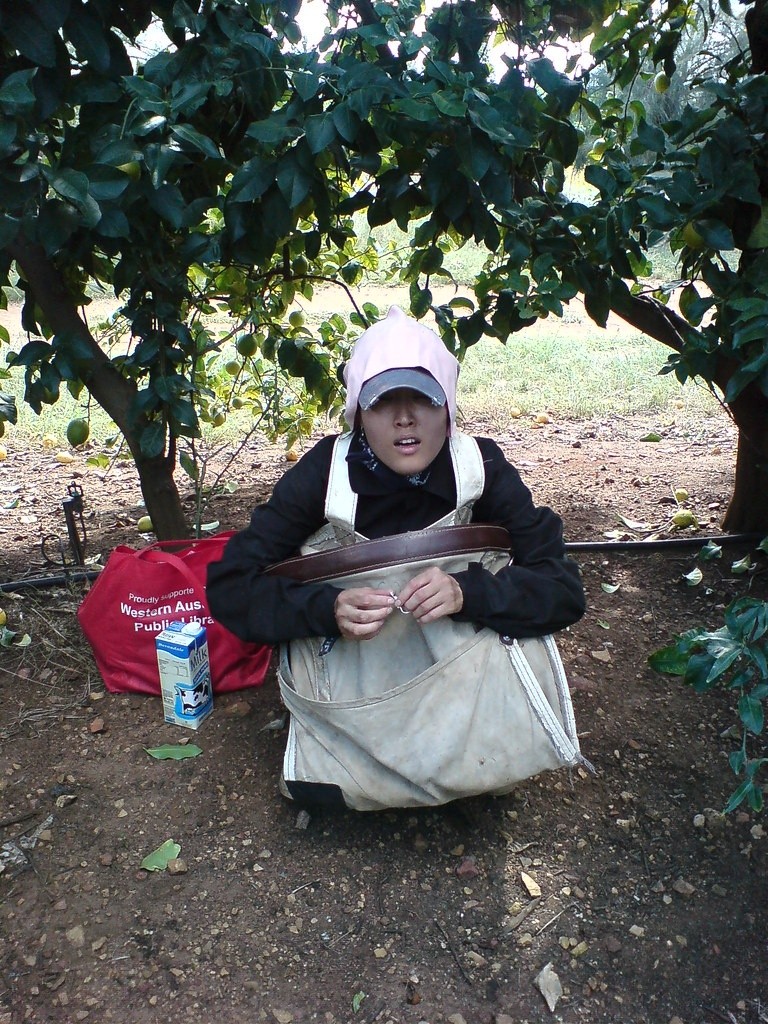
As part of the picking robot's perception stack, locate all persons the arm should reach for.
[204,305,586,808]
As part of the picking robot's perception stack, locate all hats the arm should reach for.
[357,368,446,410]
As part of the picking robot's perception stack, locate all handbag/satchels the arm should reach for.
[76,531,273,696]
[263,430,581,811]
